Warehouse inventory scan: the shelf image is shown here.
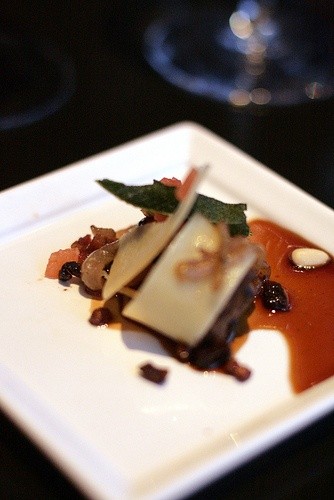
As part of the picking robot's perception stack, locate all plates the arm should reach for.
[0,119,333,498]
